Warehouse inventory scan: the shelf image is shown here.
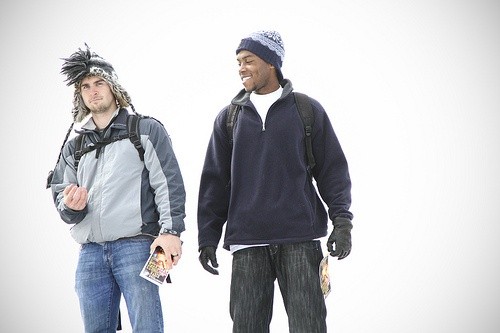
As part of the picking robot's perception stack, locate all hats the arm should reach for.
[236,29,286,81]
[46,42,173,189]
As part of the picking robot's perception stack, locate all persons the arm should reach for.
[198,30,354,333]
[46,43,187,333]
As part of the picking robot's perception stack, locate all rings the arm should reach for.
[171,254,179,257]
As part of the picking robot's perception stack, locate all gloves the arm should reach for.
[199,246,220,275]
[327,216,353,260]
